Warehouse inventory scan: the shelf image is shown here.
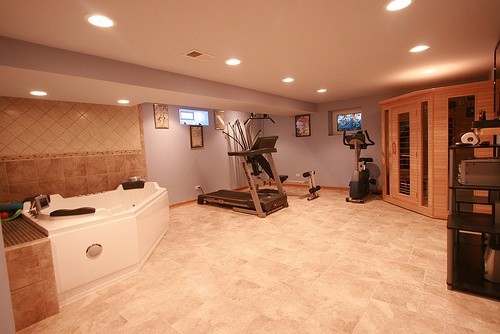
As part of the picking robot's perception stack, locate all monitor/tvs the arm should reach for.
[333,108,362,135]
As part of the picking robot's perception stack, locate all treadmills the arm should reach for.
[197,135,289,218]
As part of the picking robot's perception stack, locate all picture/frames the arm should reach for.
[295,113,311,137]
[189,125,204,148]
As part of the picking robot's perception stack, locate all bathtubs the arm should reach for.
[21,179,170,297]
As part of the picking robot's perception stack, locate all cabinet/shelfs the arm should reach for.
[379,80,500,300]
